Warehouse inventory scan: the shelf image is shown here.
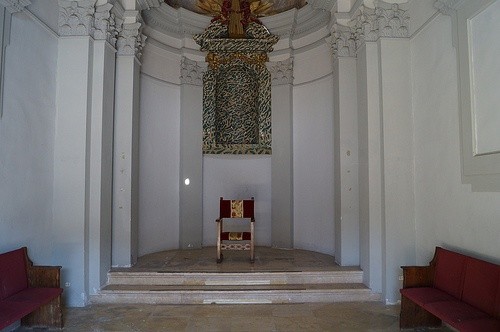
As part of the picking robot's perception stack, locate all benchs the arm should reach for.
[399,246,500,332]
[0,246,64,332]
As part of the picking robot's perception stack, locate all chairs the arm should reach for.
[216,196,256,264]
